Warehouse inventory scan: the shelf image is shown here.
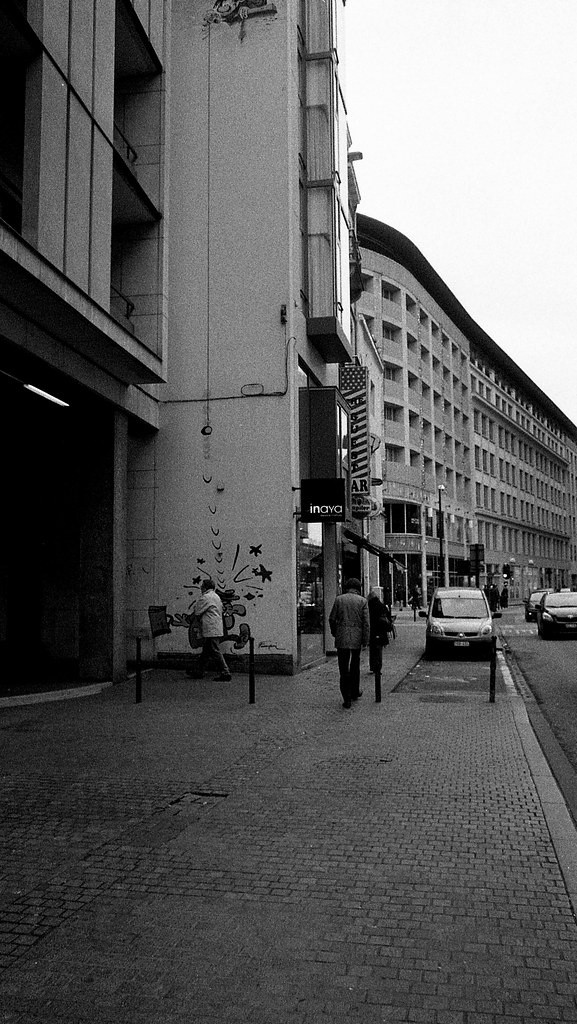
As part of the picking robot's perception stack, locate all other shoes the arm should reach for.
[370,666,374,671]
[213,673,232,682]
[352,690,363,700]
[342,699,351,708]
[187,674,203,680]
[373,669,382,675]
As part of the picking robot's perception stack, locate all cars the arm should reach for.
[418,587,502,655]
[523,588,577,640]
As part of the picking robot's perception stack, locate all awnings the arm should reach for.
[341,526,408,572]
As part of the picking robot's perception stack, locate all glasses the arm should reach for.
[201,584,207,589]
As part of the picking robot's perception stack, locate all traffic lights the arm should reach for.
[502,565,509,579]
[490,584,495,590]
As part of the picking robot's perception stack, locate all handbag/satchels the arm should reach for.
[371,602,389,648]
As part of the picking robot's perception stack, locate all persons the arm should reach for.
[329,578,370,708]
[185,579,232,682]
[366,591,392,676]
[396,583,508,613]
